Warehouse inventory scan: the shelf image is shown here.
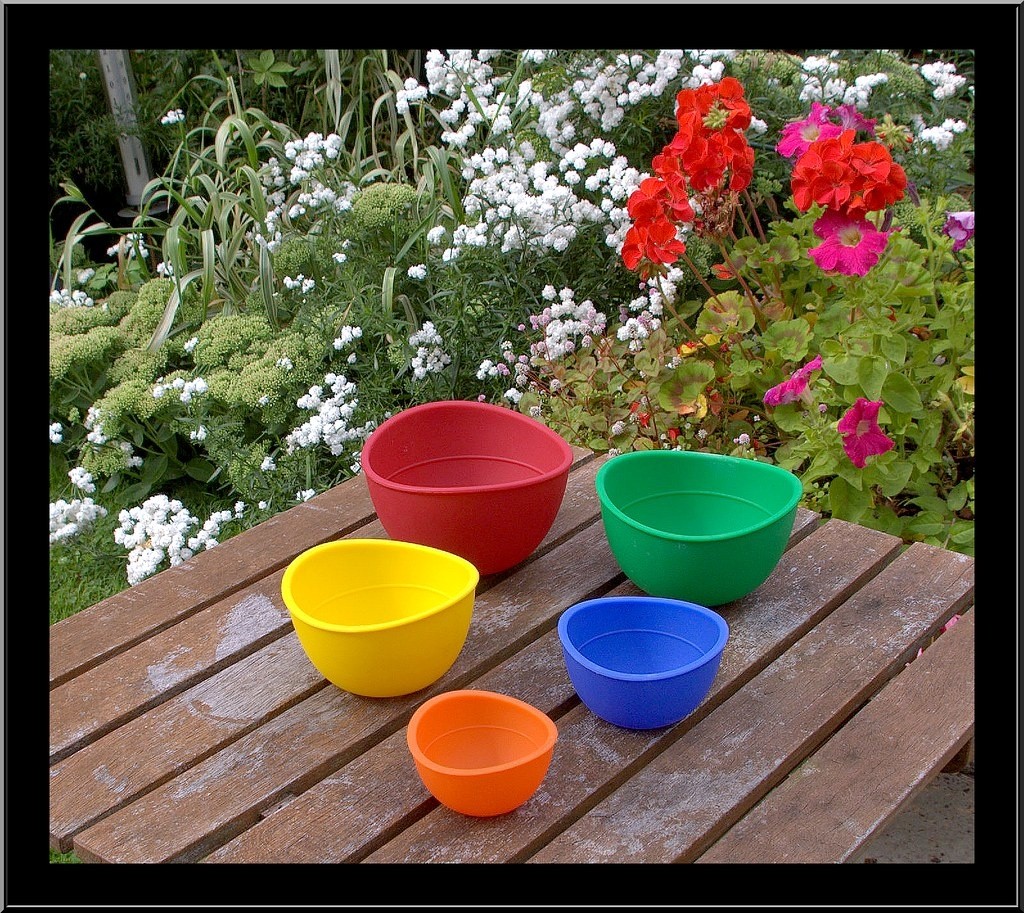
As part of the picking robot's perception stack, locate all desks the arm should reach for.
[49,443,974,864]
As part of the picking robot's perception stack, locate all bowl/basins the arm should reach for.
[408,691,560,817]
[283,538,479,698]
[559,596,730,731]
[595,450,802,604]
[361,402,573,576]
